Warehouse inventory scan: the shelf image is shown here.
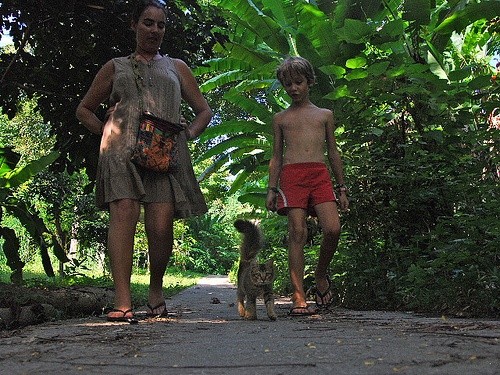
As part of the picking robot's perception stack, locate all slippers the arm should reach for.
[315,274,334,306]
[146,301,167,318]
[290,305,309,316]
[107,308,134,321]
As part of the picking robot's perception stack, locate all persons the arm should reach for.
[265,56,350,316]
[75,0,212,321]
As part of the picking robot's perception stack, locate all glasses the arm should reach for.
[152,0,166,11]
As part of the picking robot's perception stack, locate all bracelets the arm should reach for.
[337,182,348,193]
[185,128,191,139]
[98,123,104,134]
[267,187,279,194]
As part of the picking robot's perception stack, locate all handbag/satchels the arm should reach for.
[130,112,183,172]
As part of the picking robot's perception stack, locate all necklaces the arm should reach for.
[135,47,158,67]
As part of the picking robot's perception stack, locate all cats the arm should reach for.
[233,219,278,322]
